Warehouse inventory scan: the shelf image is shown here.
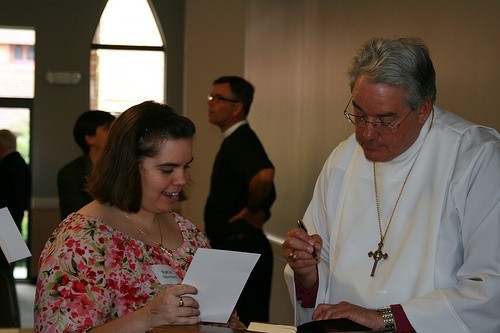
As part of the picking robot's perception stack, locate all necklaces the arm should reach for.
[125,211,173,258]
[367,107,435,277]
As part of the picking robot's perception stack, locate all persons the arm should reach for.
[282,37,500,333]
[0,128,30,330]
[32,100,247,333]
[203,75,277,328]
[57,110,117,223]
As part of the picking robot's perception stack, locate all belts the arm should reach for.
[211,232,249,241]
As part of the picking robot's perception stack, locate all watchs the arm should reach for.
[376,306,395,332]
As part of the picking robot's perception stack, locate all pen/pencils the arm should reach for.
[297,220,320,263]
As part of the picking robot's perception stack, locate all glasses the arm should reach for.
[207,95,240,103]
[343,95,414,135]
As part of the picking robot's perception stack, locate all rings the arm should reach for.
[179,297,183,307]
[289,249,297,261]
